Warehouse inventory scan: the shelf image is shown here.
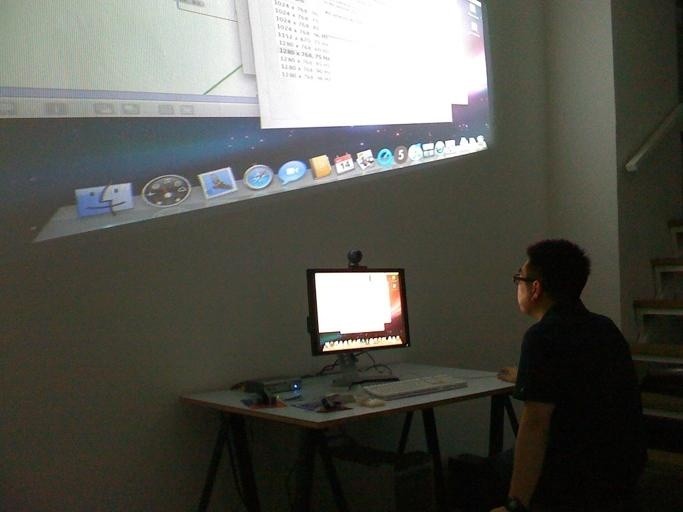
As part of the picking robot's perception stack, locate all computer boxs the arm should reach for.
[306,446,435,512]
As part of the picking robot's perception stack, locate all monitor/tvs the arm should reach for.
[307,267,411,387]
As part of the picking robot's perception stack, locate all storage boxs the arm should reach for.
[265,434,437,512]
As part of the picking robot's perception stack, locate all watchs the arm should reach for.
[505,496,528,512]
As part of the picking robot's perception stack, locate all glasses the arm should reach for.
[513,272,533,286]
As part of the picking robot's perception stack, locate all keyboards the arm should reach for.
[361,372,468,402]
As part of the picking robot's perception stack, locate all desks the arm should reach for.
[181,364,520,512]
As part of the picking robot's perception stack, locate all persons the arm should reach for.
[450,239,649,511]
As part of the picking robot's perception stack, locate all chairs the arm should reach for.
[528,369,648,512]
